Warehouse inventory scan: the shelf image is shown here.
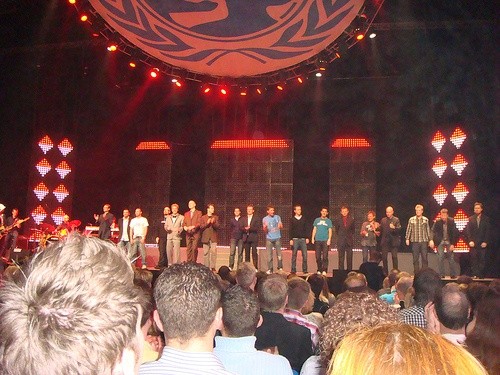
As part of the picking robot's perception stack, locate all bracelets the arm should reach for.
[328,239,332,242]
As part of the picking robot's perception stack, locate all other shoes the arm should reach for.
[440,275,445,278]
[266,270,273,274]
[318,271,321,274]
[322,271,326,276]
[451,275,457,279]
[212,268,215,271]
[142,264,146,269]
[155,265,160,269]
[228,266,232,272]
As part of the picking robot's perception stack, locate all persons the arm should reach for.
[288,204,310,275]
[227,207,243,272]
[93,204,116,239]
[164,203,185,269]
[360,210,381,264]
[138,259,236,375]
[239,204,259,273]
[300,261,500,375]
[369,206,402,276]
[262,206,284,275]
[153,206,171,269]
[428,208,460,280]
[334,206,355,272]
[117,208,133,266]
[405,204,435,276]
[0,227,153,375]
[0,203,24,262]
[0,261,337,375]
[311,207,332,276]
[465,202,490,279]
[128,207,149,270]
[199,204,220,272]
[182,200,202,265]
